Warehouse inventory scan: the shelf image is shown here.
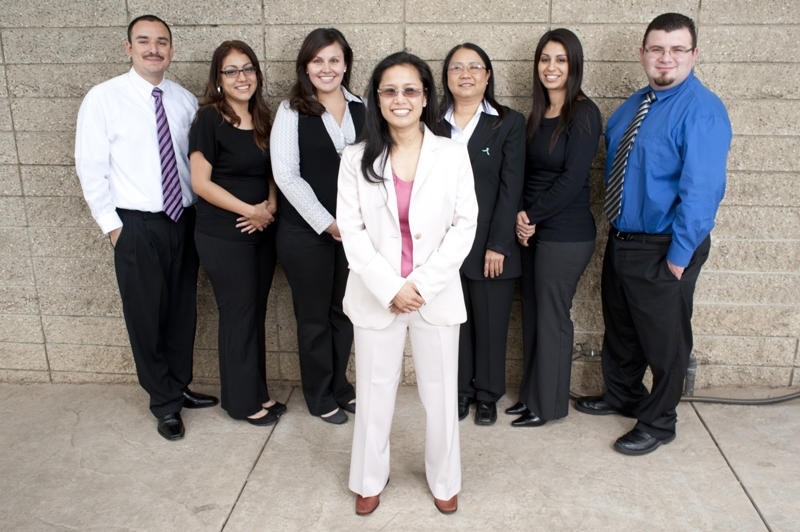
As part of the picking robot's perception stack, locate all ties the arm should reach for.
[151,87,184,223]
[603,89,657,223]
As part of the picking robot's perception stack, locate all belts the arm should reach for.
[610,228,672,241]
[117,208,194,219]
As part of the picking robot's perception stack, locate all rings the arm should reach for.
[518,233,521,236]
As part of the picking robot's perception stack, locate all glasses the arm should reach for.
[642,44,693,57]
[220,62,258,78]
[376,85,427,98]
[448,62,487,74]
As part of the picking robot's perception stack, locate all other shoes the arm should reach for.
[246,407,279,426]
[355,478,389,514]
[341,401,356,413]
[505,399,527,413]
[262,401,287,413]
[434,494,457,513]
[320,407,348,424]
[511,410,543,427]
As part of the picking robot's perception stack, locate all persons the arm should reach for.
[440,42,526,426]
[336,52,478,516]
[269,28,367,425]
[75,15,219,442]
[574,13,732,456]
[505,28,602,426]
[187,40,287,426]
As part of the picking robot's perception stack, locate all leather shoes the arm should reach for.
[475,399,498,425]
[157,411,185,441]
[458,394,476,420]
[180,387,219,409]
[614,429,676,454]
[576,394,620,415]
